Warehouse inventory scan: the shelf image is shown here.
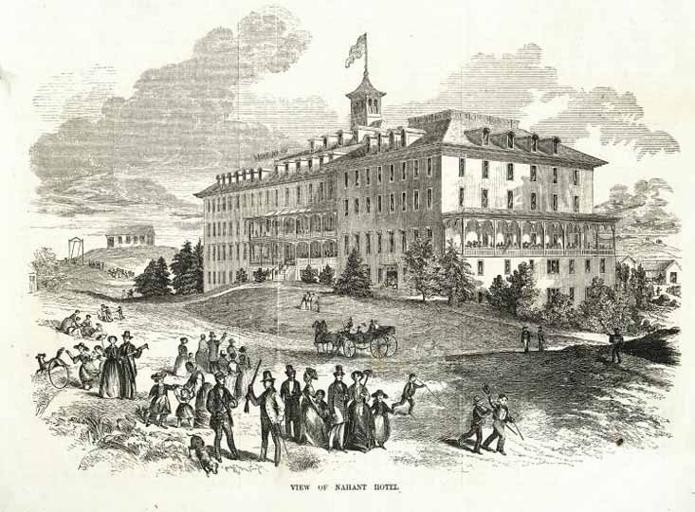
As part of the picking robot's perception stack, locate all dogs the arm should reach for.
[35,347,72,375]
[186,433,219,478]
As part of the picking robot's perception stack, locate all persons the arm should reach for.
[483,393,516,457]
[609,327,625,365]
[458,395,492,455]
[58,287,426,475]
[537,324,545,350]
[519,324,531,354]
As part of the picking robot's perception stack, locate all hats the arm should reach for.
[334,364,345,377]
[372,390,388,399]
[285,365,296,374]
[107,336,117,343]
[350,371,364,381]
[472,395,484,406]
[215,371,227,379]
[151,372,167,379]
[122,331,133,339]
[73,342,89,351]
[209,332,216,337]
[260,371,276,382]
[220,338,248,358]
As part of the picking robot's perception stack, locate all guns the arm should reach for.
[245,360,261,413]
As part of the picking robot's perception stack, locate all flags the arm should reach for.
[344,34,365,68]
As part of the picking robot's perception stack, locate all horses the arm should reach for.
[312,320,344,356]
